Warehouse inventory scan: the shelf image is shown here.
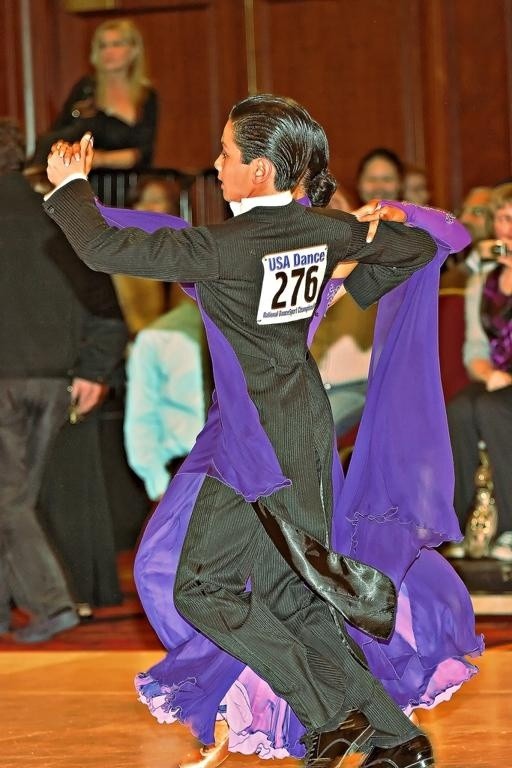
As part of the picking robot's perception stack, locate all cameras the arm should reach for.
[490,243,507,256]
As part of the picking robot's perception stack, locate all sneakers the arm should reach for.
[440,526,466,562]
[175,733,235,768]
[490,528,512,563]
[9,605,86,647]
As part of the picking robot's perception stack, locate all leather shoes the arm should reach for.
[300,707,377,768]
[355,733,436,768]
